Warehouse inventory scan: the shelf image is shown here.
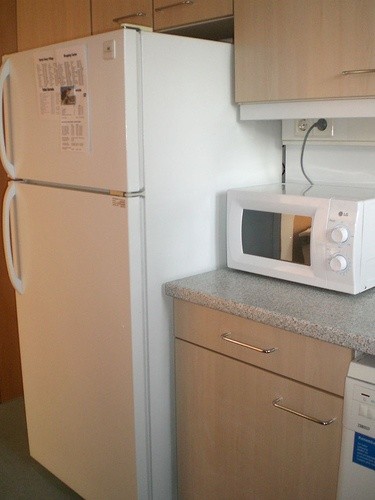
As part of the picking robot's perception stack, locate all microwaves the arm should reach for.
[226,182,375,295]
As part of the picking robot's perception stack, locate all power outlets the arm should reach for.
[295,119,333,137]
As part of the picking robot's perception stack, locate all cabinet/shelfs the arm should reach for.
[16,0,91,52]
[173,299,356,500]
[91,0,233,34]
[234,0,375,103]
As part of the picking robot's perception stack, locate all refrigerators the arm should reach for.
[0,23,287,499]
